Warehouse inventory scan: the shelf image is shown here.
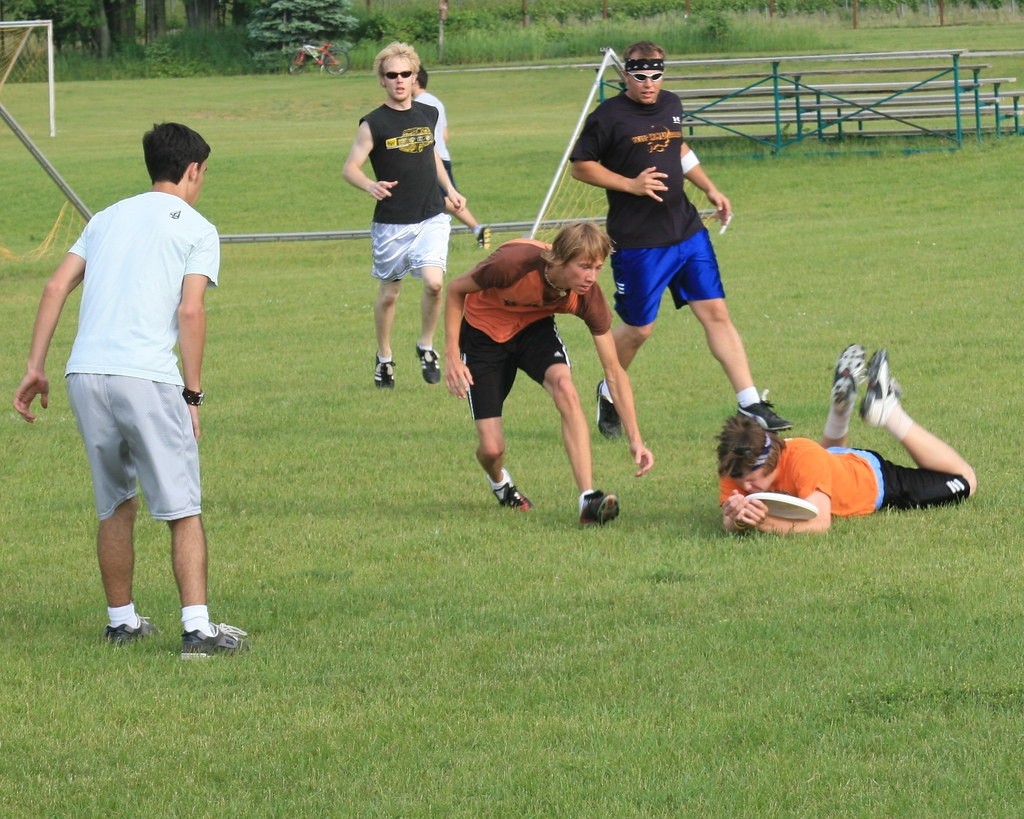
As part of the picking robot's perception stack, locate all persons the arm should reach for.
[12,122,252,658]
[716,342,978,534]
[570,41,795,441]
[444,221,655,526]
[342,42,490,391]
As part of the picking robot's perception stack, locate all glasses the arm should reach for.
[384,71,412,79]
[627,72,663,82]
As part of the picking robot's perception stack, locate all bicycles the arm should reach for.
[288,35,351,75]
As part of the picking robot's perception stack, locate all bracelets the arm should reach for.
[733,520,749,531]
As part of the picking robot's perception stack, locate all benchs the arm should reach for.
[594,48,1024,159]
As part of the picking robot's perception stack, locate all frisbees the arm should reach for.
[745,492,819,521]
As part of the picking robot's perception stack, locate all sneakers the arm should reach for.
[416,341,441,384]
[596,381,622,440]
[737,400,794,431]
[830,343,869,415]
[478,227,493,250]
[493,482,533,513]
[579,490,619,526]
[859,348,903,427]
[103,613,160,645]
[181,622,250,660]
[374,351,396,390]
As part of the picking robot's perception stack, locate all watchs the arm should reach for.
[182,388,205,407]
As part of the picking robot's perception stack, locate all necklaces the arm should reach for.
[544,262,567,298]
